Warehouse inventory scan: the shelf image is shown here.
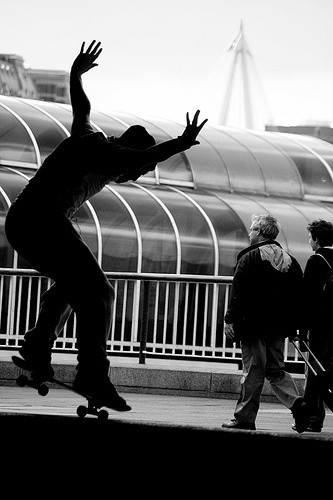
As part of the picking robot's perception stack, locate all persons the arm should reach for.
[4,39,209,411]
[222,214,311,431]
[292,218,333,432]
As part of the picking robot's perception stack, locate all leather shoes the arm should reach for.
[292,424,321,432]
[292,401,309,433]
[222,418,256,430]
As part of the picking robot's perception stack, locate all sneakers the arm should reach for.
[18,342,56,378]
[70,374,126,407]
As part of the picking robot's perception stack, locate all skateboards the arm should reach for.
[11,355,132,421]
